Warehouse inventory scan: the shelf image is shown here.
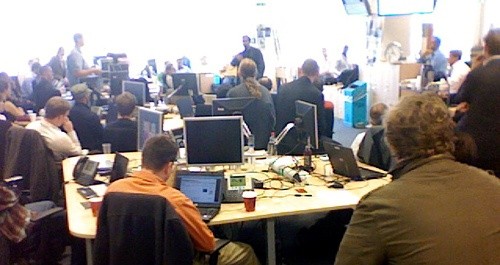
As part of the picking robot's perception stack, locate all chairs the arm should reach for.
[93,192,221,265]
[2,126,62,202]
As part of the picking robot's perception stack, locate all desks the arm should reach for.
[62,146,393,265]
[322,85,344,120]
[370,62,418,108]
[99,113,183,131]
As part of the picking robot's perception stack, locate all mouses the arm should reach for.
[326,182,343,189]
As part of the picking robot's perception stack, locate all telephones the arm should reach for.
[73,157,99,185]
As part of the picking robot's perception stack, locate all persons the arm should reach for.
[227,58,276,151]
[276,59,334,156]
[232,36,265,79]
[351,103,397,171]
[0,34,138,163]
[416,27,500,179]
[335,92,500,265]
[107,135,260,265]
[140,58,190,94]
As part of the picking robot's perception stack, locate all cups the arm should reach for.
[242,191,258,212]
[102,143,111,156]
[89,197,102,217]
[30,113,36,121]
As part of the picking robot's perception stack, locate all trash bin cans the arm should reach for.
[344,80,366,128]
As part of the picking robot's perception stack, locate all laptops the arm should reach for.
[77,151,129,199]
[172,171,224,221]
[324,142,387,181]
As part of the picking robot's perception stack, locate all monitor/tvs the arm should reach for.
[342,0,437,16]
[109,70,318,167]
